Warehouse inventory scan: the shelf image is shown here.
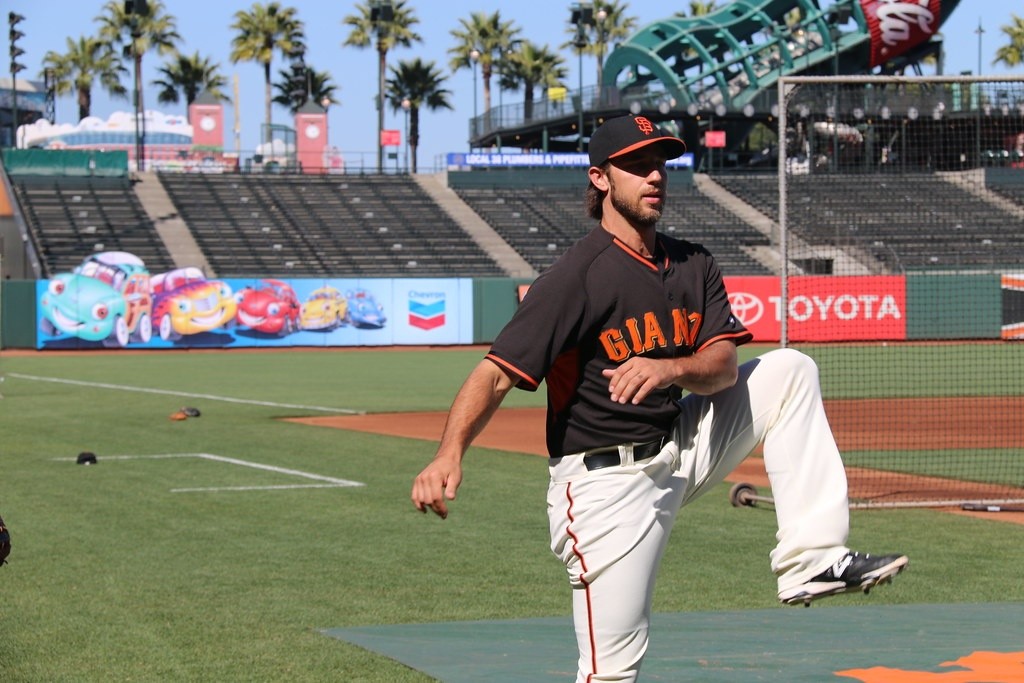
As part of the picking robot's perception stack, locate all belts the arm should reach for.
[583,433,667,471]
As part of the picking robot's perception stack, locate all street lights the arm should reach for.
[321,98,330,143]
[470,50,480,147]
[595,10,609,88]
[401,99,413,175]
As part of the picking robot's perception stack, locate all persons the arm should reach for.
[411,118,908,683]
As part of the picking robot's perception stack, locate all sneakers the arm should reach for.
[778,552,909,609]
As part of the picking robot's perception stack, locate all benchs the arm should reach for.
[10,164,1024,282]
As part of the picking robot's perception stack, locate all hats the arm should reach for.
[588,115,686,167]
